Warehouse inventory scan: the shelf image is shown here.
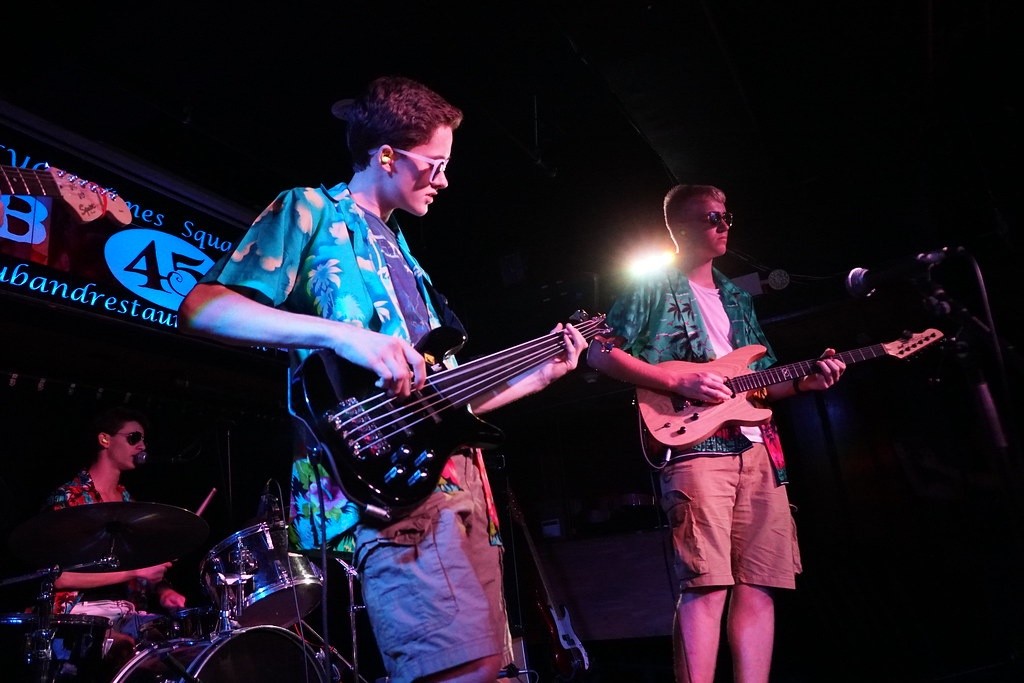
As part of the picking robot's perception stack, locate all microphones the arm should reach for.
[137,451,173,466]
[257,478,272,519]
[846,267,945,305]
[916,252,975,267]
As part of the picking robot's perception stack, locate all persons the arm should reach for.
[43,405,236,683]
[179,79,587,683]
[587,185,847,683]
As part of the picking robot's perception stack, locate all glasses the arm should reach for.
[681,211,733,227]
[369,147,449,183]
[109,431,149,446]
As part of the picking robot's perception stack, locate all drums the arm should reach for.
[0,612,115,683]
[138,605,232,650]
[200,519,324,625]
[112,625,339,682]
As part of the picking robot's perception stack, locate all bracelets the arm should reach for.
[793,378,801,393]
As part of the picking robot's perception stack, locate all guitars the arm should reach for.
[489,452,592,683]
[287,307,628,531]
[632,327,958,458]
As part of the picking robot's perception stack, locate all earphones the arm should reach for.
[382,155,390,163]
[102,437,108,443]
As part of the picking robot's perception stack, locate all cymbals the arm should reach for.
[42,500,210,559]
